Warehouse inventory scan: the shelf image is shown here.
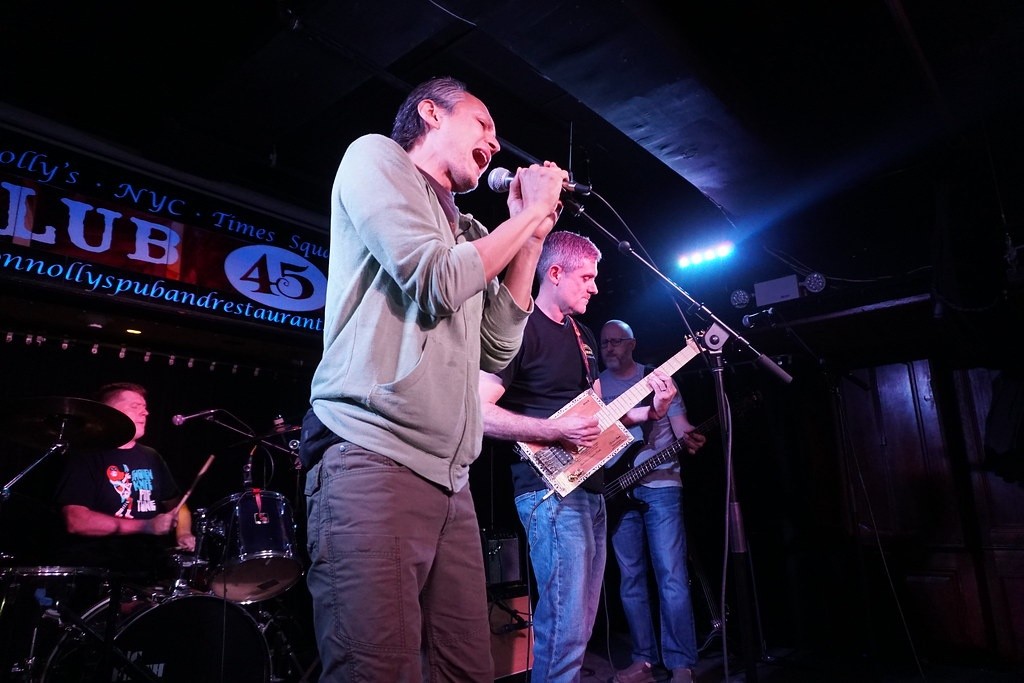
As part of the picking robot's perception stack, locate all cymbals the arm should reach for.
[0,393,138,453]
[229,423,301,449]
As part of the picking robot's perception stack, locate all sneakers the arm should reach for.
[613,658,668,683]
[671,667,695,683]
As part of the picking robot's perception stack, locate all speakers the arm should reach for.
[488,587,535,681]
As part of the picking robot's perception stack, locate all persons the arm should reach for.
[483,231,677,683]
[299,77,569,683]
[60,382,197,580]
[593,320,706,683]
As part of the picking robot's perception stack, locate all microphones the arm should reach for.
[487,166,591,193]
[742,308,777,328]
[172,409,217,426]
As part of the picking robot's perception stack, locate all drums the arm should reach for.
[189,487,306,603]
[0,565,112,672]
[41,583,274,683]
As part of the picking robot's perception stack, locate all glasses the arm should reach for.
[599,338,631,348]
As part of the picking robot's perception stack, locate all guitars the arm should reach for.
[596,388,765,497]
[516,328,707,496]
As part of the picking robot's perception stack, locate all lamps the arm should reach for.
[730,271,826,310]
